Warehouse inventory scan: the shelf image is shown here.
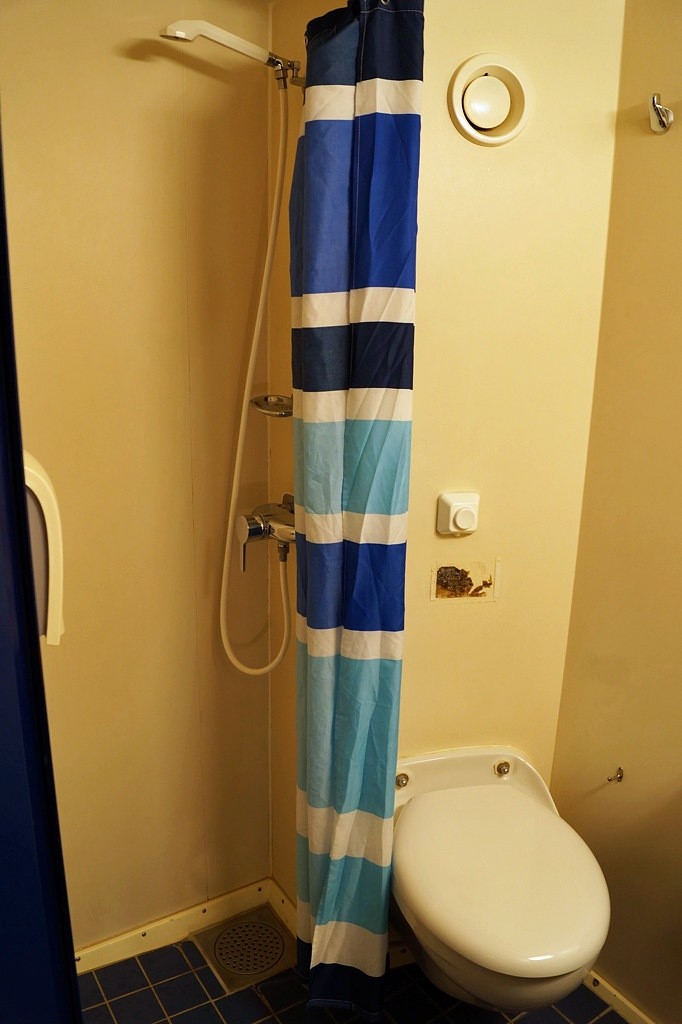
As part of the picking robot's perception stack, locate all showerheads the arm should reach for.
[158,19,272,65]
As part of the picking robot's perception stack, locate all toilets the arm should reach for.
[389,741,611,1013]
